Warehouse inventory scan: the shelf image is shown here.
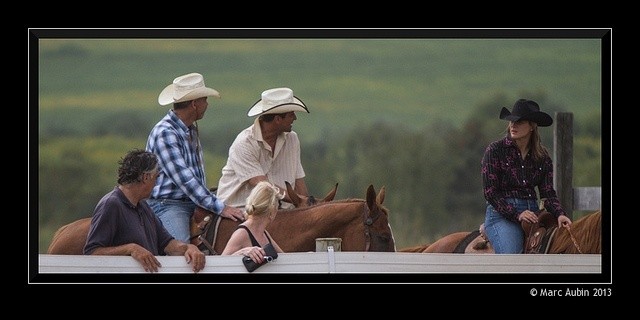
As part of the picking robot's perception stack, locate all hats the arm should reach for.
[158,73,221,105]
[247,88,310,117]
[499,99,553,128]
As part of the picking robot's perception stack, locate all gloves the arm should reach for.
[242,242,279,273]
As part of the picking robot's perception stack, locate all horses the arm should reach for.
[210,181,339,209]
[397,210,602,254]
[46,184,397,255]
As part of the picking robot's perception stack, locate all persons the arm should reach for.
[82,148,206,274]
[144,72,246,252]
[216,86,312,213]
[482,98,572,254]
[219,180,284,256]
